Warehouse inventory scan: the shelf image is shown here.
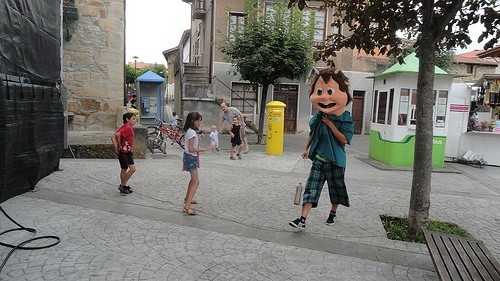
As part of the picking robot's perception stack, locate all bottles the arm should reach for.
[294,182,303,205]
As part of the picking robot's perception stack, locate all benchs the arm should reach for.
[422,227,500,281]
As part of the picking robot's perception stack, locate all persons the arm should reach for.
[111,112,137,194]
[219,103,249,154]
[170,112,179,131]
[130,94,137,109]
[228,117,243,161]
[181,112,205,215]
[288,68,355,230]
[210,125,220,151]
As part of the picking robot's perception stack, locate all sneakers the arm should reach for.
[325,214,337,225]
[289,218,305,230]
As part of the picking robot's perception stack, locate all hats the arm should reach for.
[232,118,239,122]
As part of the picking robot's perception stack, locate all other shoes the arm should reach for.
[212,148,214,151]
[236,153,241,159]
[227,150,236,153]
[215,147,219,151]
[244,148,249,154]
[230,156,235,160]
[118,184,132,194]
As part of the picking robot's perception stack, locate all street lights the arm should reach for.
[132,56,139,75]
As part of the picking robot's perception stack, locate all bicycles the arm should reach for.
[145,113,202,151]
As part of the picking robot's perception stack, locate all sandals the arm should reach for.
[184,198,199,204]
[181,207,198,215]
